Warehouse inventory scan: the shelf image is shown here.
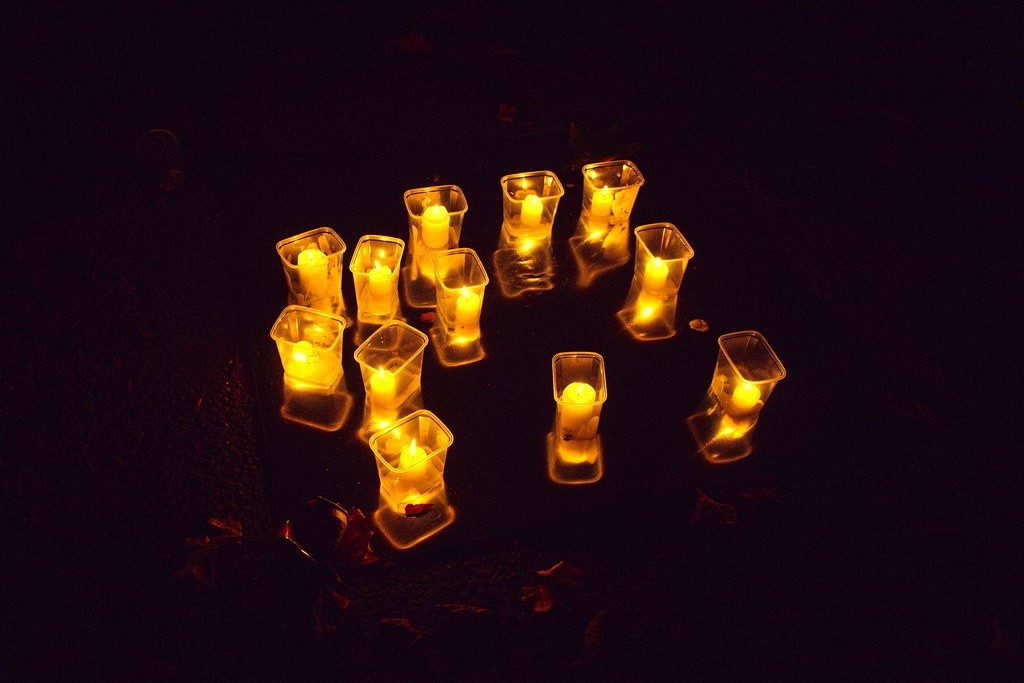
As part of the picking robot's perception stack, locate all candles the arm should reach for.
[371,365,396,407]
[591,186,613,216]
[369,261,392,297]
[562,382,596,431]
[398,440,427,478]
[422,205,450,248]
[521,194,543,225]
[293,340,313,362]
[732,382,761,409]
[298,249,329,293]
[456,286,480,323]
[644,257,669,289]
